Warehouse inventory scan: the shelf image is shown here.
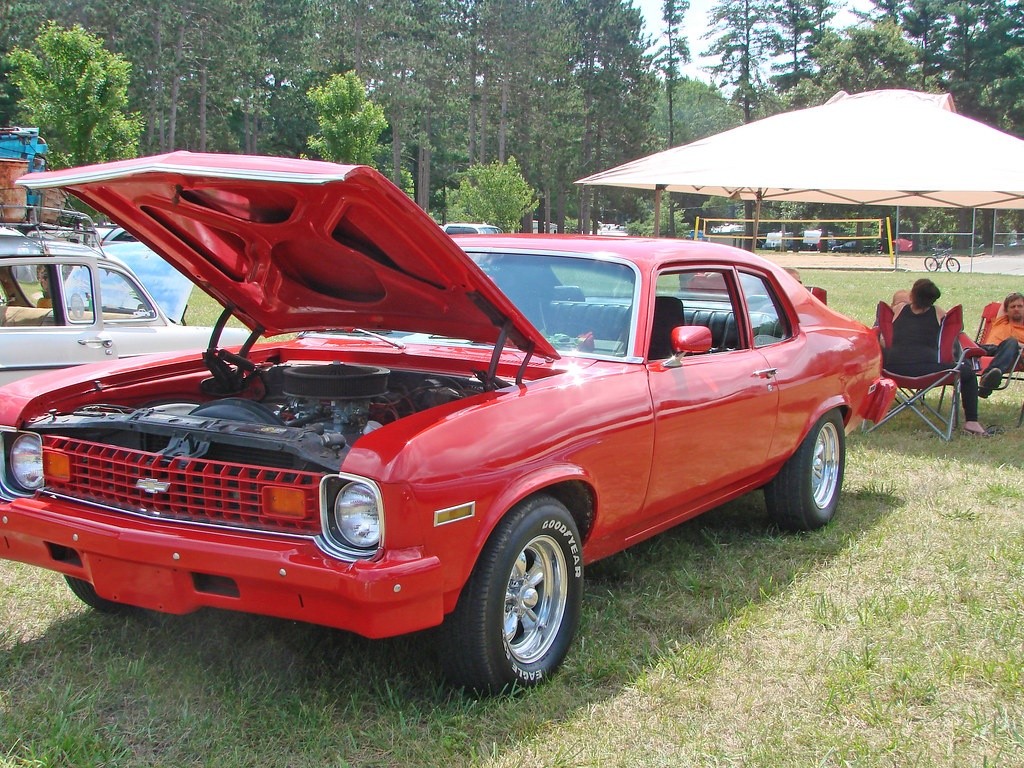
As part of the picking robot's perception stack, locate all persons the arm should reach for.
[872,278,1024,436]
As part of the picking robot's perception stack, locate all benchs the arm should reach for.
[0,306,150,327]
[541,301,782,356]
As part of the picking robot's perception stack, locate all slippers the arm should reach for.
[963,427,990,437]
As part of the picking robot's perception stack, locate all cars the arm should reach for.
[441,221,507,266]
[1,232,257,394]
[832,240,875,254]
[1,148,896,702]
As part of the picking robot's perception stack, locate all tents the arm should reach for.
[572,89,1024,270]
[880,238,913,252]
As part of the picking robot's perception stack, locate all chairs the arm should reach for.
[972,302,1024,429]
[861,301,986,444]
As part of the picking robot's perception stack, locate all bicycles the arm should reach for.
[924,248,961,273]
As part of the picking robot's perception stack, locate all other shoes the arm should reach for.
[977,368,1002,399]
